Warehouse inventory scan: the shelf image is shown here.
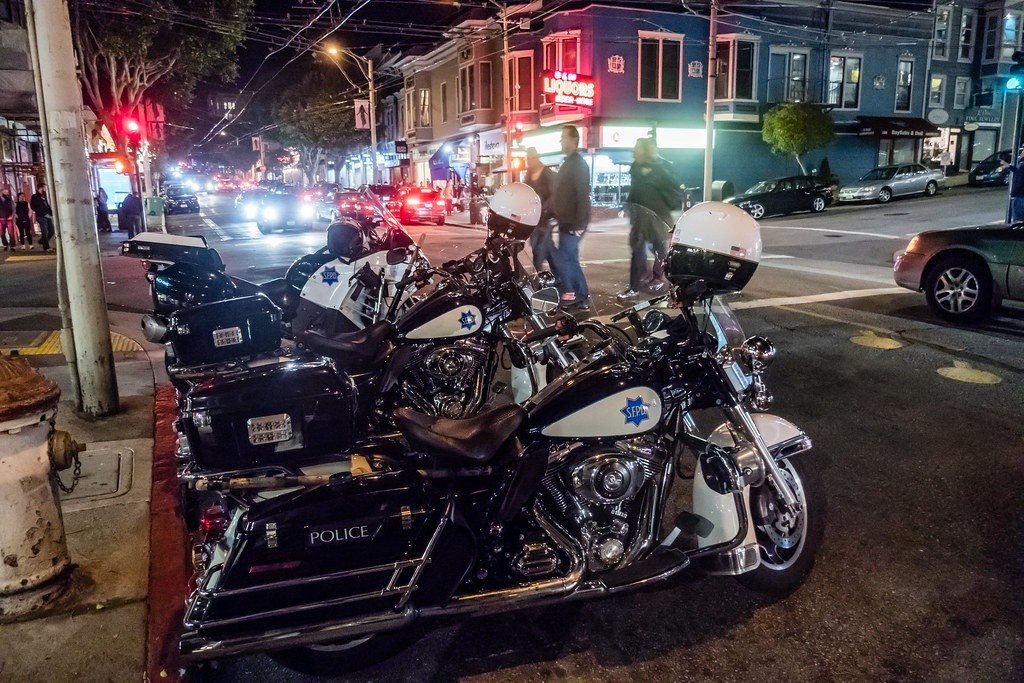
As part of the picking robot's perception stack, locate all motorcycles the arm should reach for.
[116,188,824,677]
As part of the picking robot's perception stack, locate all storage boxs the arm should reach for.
[120,232,206,258]
[168,293,282,363]
[182,357,359,471]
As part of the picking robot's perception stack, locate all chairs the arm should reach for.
[885,172,890,175]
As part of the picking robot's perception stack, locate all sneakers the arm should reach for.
[617,289,640,299]
[649,278,663,286]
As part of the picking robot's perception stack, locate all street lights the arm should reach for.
[328,46,377,181]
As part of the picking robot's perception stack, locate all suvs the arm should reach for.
[357,184,402,218]
[386,186,446,225]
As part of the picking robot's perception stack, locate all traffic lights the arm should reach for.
[1005,51,1023,91]
[125,120,142,143]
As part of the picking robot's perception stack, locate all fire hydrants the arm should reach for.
[1,350,89,623]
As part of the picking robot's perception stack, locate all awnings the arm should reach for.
[857,115,941,137]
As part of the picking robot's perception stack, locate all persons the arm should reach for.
[356,104,368,128]
[383,179,416,189]
[819,156,829,175]
[548,124,591,308]
[521,147,558,279]
[12,193,35,250]
[123,192,142,238]
[470,182,479,198]
[0,189,16,250]
[616,136,683,299]
[443,178,465,216]
[1001,150,1024,222]
[94,187,112,233]
[29,183,54,252]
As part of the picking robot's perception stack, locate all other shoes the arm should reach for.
[21,245,26,249]
[46,248,54,252]
[4,246,8,251]
[38,239,43,244]
[29,244,34,250]
[11,248,16,252]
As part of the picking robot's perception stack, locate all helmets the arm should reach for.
[664,201,761,295]
[326,216,369,257]
[490,182,542,226]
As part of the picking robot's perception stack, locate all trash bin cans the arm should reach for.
[115,202,129,230]
[470,197,490,225]
[684,187,701,212]
[146,197,162,216]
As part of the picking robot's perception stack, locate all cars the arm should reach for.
[894,223,1024,320]
[967,148,1023,187]
[169,168,374,235]
[722,176,834,221]
[164,180,200,215]
[838,163,945,202]
